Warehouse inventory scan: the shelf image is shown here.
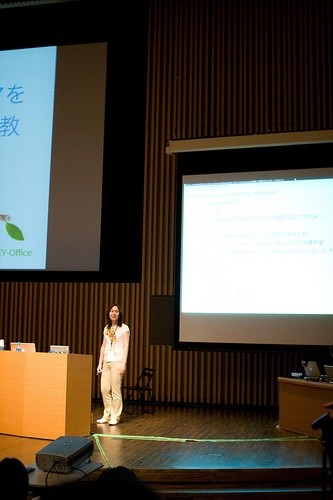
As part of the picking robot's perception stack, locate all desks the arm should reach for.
[277,376,333,438]
[0,350,93,440]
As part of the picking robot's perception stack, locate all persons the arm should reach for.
[0,458,159,500]
[97,304,130,425]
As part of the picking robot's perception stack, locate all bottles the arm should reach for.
[16,342,21,351]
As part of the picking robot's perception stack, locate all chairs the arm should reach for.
[131,368,155,416]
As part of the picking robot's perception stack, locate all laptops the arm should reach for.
[50,345,70,354]
[324,366,333,381]
[301,361,326,377]
[11,343,36,353]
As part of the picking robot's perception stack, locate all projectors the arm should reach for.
[36,435,94,473]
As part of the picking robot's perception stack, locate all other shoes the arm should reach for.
[110,419,119,425]
[96,416,109,424]
